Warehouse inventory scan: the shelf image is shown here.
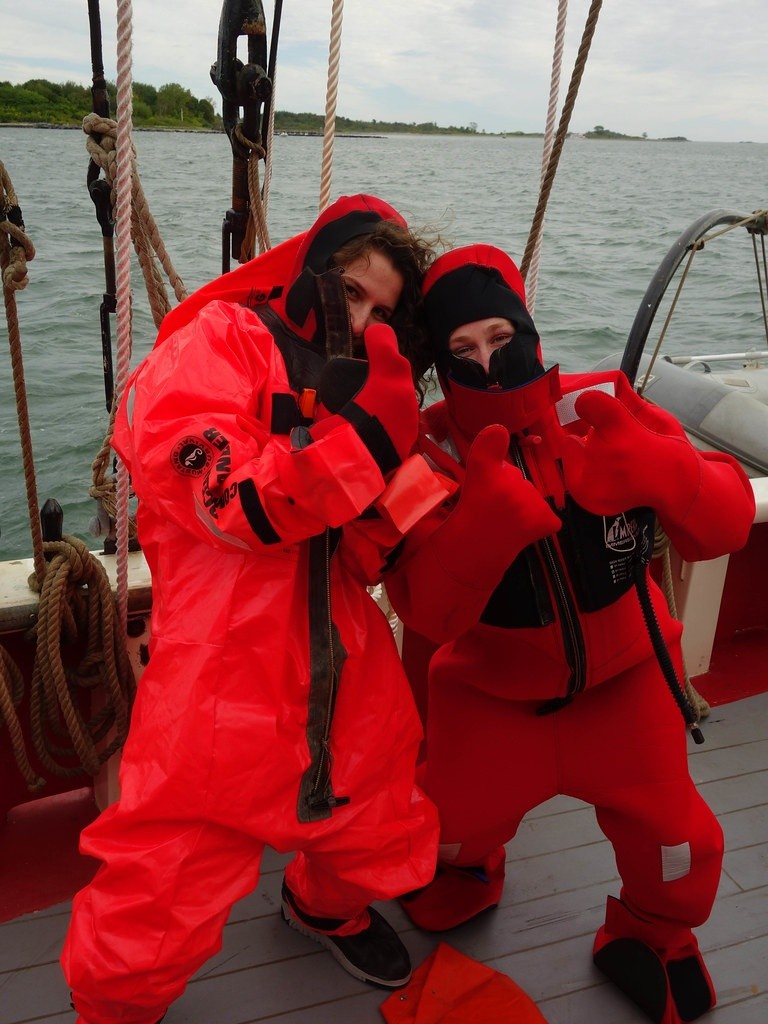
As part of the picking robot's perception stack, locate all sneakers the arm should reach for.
[280,886,413,991]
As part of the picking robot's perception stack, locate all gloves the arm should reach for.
[429,423,561,593]
[307,322,418,516]
[564,390,750,559]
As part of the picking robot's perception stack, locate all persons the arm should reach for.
[62,196,442,1024]
[385,244,755,1024]
